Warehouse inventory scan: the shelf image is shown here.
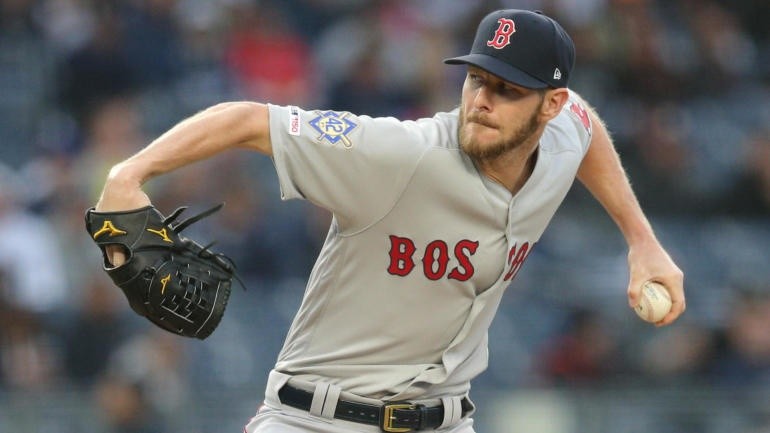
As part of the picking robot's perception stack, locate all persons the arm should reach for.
[83,10,685,433]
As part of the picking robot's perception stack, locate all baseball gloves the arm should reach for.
[85,204,230,342]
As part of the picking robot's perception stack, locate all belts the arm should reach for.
[280,383,474,433]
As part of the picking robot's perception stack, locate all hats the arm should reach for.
[442,9,576,90]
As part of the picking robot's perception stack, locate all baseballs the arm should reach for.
[634,282,672,324]
